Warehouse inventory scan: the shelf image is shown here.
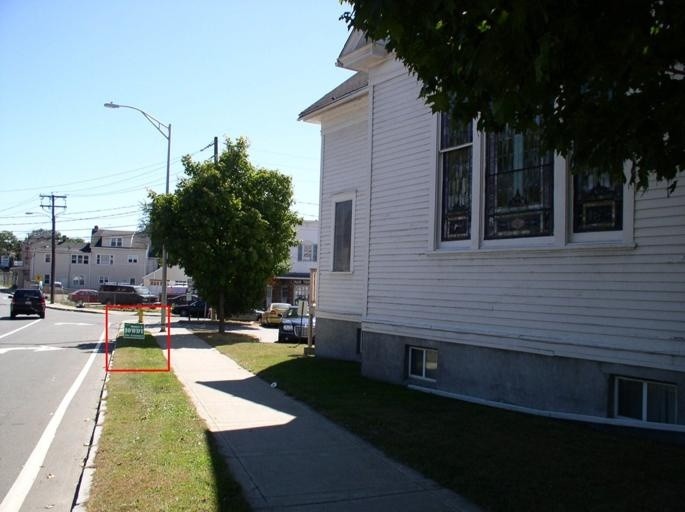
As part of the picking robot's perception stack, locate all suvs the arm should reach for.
[48,281,64,293]
[7,288,49,319]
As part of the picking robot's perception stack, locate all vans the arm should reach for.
[99,283,160,305]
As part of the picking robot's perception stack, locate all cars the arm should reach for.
[172,299,212,318]
[168,295,199,305]
[278,306,317,344]
[207,306,265,322]
[28,280,42,289]
[68,289,100,304]
[261,303,295,326]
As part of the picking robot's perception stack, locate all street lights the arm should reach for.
[101,98,172,333]
[24,210,54,302]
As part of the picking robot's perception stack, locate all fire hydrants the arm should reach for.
[135,306,144,324]
